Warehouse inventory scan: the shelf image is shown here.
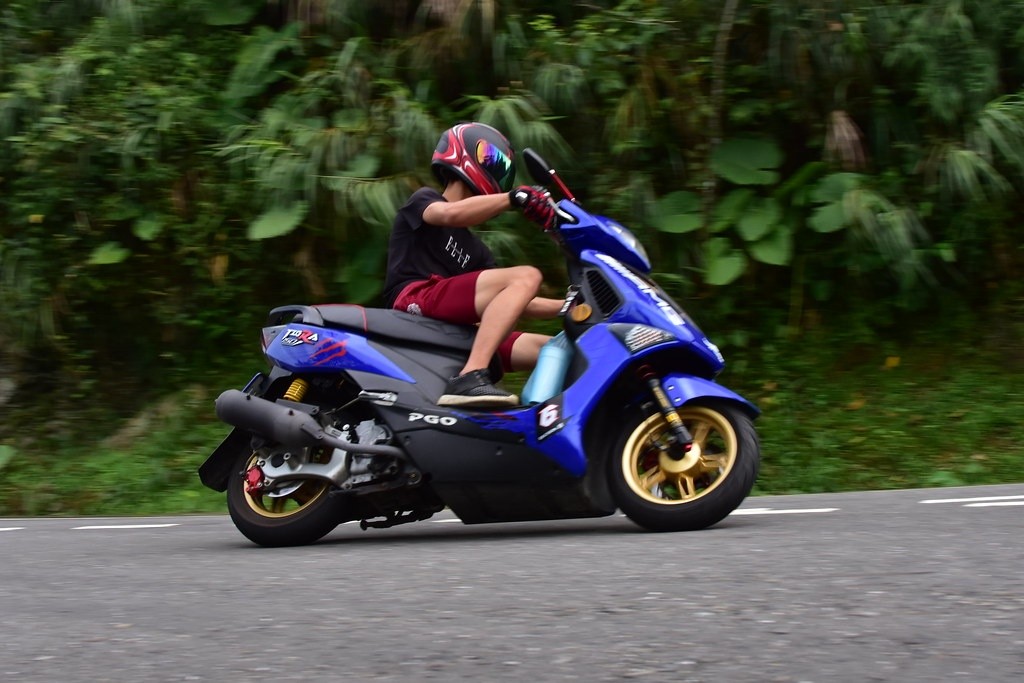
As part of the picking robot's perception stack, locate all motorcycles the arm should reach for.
[197,147,763,548]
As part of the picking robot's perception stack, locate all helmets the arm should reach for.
[430,122,517,195]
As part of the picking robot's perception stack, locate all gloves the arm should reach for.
[557,285,585,318]
[509,184,559,232]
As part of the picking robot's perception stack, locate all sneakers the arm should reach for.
[436,368,519,406]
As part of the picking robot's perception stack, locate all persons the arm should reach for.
[390,121,579,407]
